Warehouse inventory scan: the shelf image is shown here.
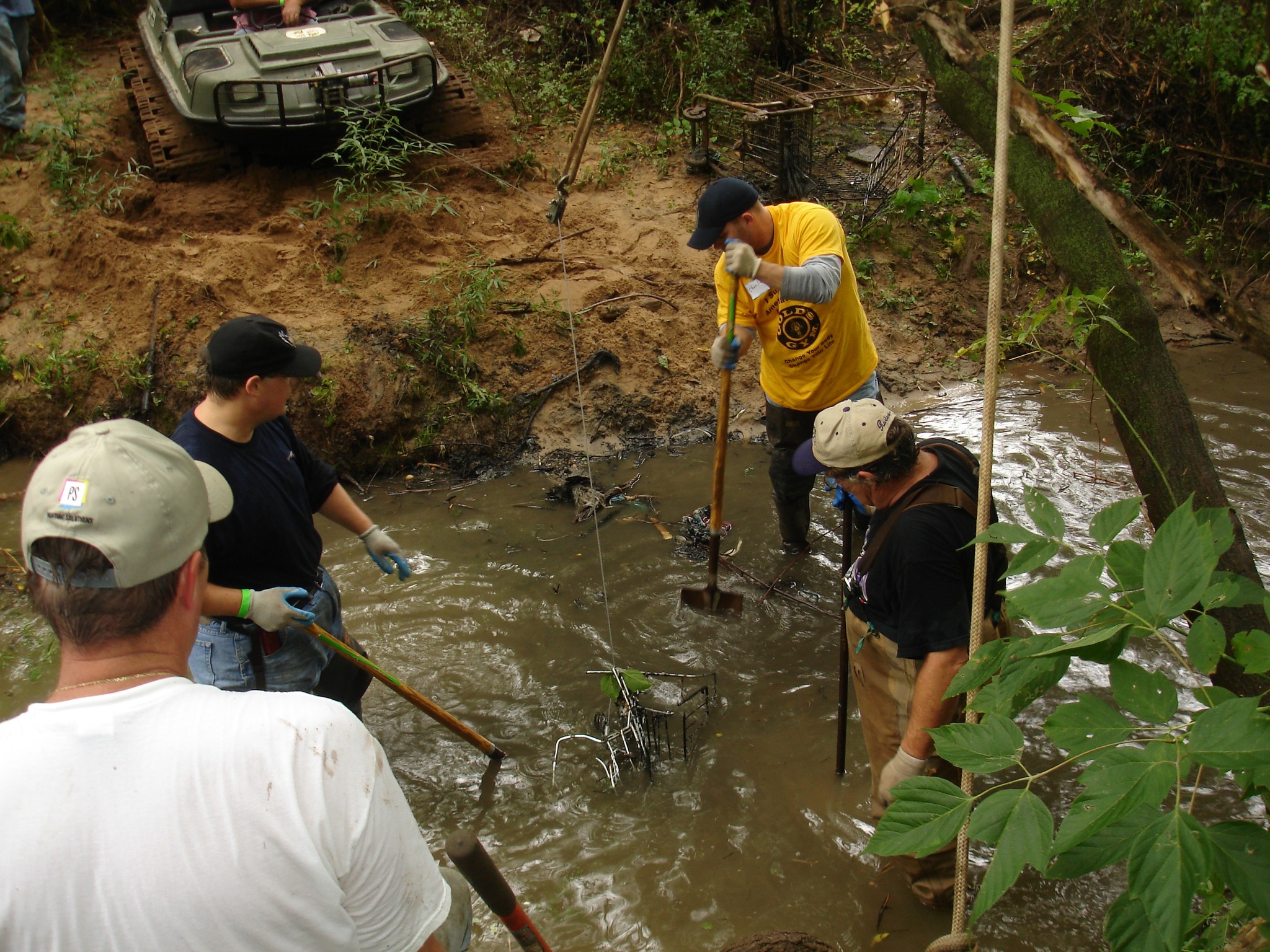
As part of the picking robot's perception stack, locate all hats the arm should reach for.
[792,400,906,475]
[21,418,234,592]
[204,314,321,378]
[687,176,759,250]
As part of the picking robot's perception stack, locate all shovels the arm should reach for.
[681,276,743,617]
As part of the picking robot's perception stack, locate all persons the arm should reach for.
[171,316,415,699]
[687,174,879,547]
[231,0,321,37]
[1,413,471,951]
[794,396,1011,895]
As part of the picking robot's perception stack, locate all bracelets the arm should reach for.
[280,0,284,6]
[238,588,250,620]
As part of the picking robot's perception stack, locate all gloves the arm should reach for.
[877,745,929,809]
[825,480,878,516]
[724,236,764,280]
[710,326,741,371]
[238,588,317,633]
[360,526,412,582]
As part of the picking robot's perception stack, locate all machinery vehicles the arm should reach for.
[115,0,486,187]
